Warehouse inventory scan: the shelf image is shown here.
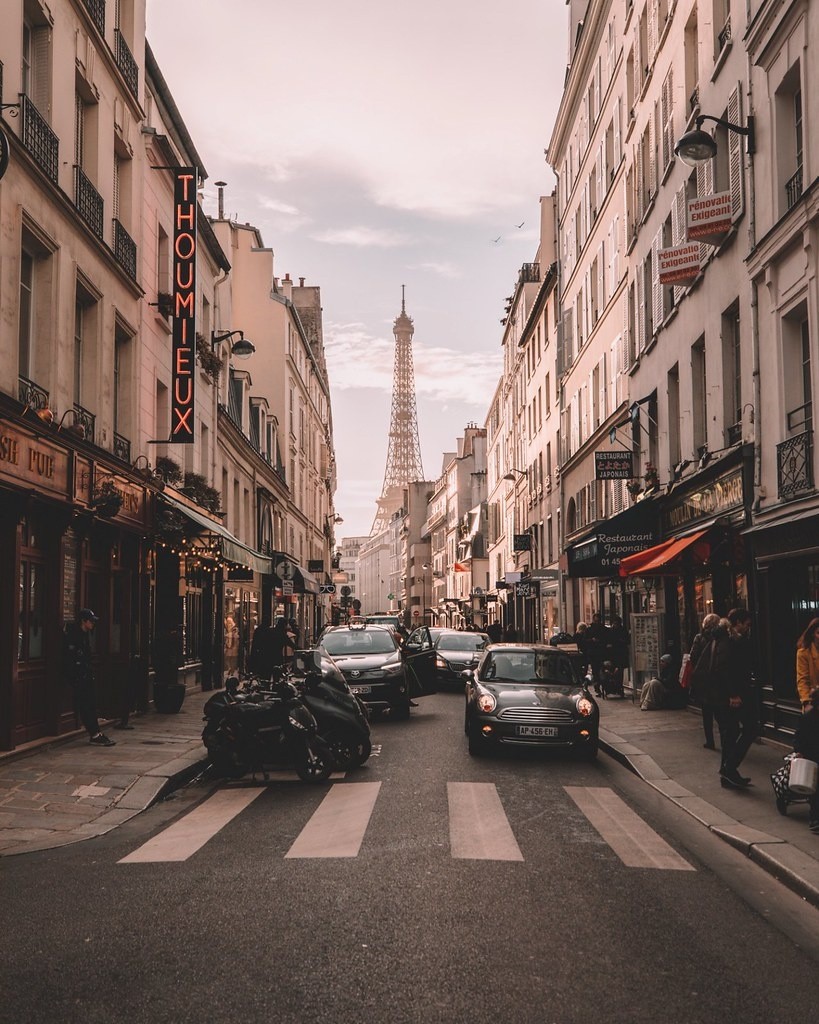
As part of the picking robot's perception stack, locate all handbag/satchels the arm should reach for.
[679,653,693,689]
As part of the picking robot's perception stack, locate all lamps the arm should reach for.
[124,455,156,480]
[627,576,636,592]
[665,471,697,496]
[19,390,54,428]
[331,549,342,558]
[675,457,718,473]
[211,330,255,360]
[643,483,667,495]
[503,468,529,481]
[55,409,86,440]
[606,578,619,594]
[698,444,742,469]
[141,466,166,493]
[675,112,756,167]
[328,512,344,526]
[421,561,433,570]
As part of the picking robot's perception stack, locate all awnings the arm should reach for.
[160,492,273,574]
[272,557,319,594]
[629,526,710,574]
[563,504,662,578]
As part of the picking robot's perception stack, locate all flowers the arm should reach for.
[89,480,124,508]
[626,480,640,493]
[643,463,658,482]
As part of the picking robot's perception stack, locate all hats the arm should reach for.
[660,654,671,663]
[78,608,100,622]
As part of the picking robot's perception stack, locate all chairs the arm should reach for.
[494,656,512,679]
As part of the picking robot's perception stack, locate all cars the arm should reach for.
[461,642,600,762]
[310,624,438,722]
[365,615,492,692]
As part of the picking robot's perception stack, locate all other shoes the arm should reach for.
[720,775,751,787]
[808,810,819,831]
[89,733,116,746]
[596,691,602,696]
[703,741,714,749]
[718,765,746,787]
[754,738,765,745]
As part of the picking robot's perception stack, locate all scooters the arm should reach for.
[201,646,371,783]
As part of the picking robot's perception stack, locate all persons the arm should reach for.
[573,614,630,697]
[458,620,516,643]
[691,608,774,789]
[67,610,115,745]
[248,617,300,674]
[795,617,819,834]
[640,654,689,710]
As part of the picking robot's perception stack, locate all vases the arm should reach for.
[631,491,639,501]
[645,478,657,487]
[96,502,120,518]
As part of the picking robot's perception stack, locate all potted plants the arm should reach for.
[158,291,173,321]
[148,632,188,715]
